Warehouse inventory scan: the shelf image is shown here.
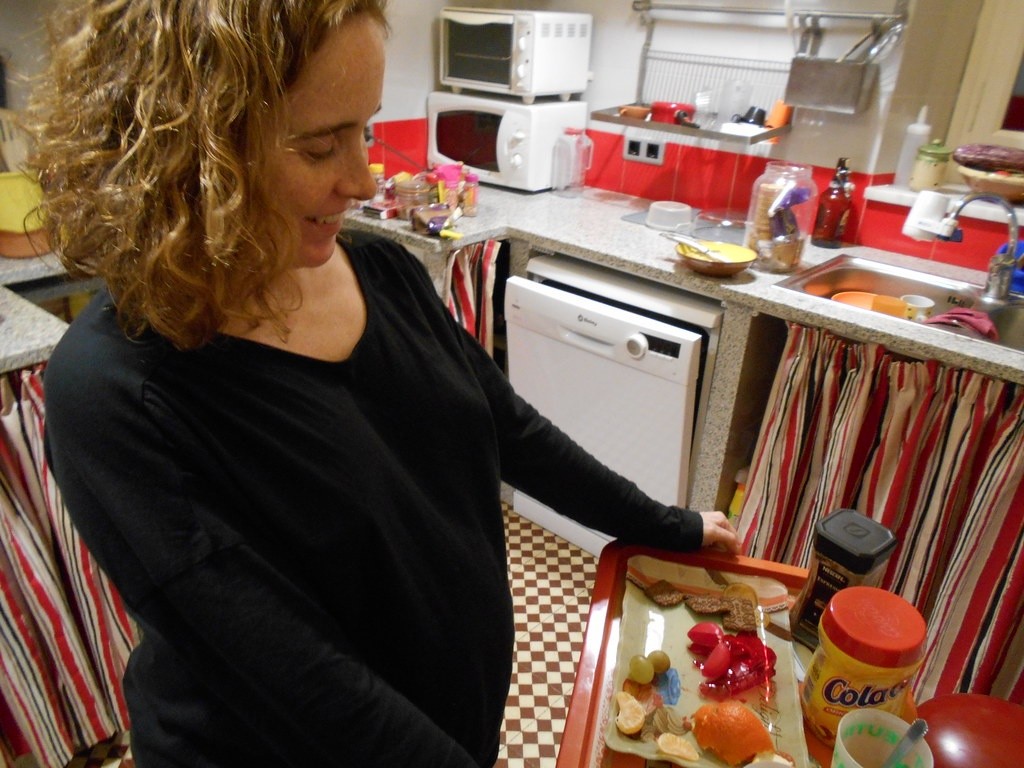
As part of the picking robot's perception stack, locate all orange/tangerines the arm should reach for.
[613,691,645,734]
[656,733,700,761]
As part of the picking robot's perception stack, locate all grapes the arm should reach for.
[628,650,670,684]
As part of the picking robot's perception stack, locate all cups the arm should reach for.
[870,295,907,318]
[744,107,766,125]
[900,294,936,325]
[830,707,935,768]
[908,139,952,194]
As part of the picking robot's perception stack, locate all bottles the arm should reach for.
[550,127,593,201]
[395,180,430,220]
[892,105,933,187]
[810,157,854,250]
[740,162,817,274]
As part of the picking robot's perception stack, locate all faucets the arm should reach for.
[900,191,1018,300]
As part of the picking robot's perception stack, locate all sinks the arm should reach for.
[966,294,1024,354]
[772,252,978,332]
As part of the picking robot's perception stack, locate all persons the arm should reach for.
[35,0,744,768]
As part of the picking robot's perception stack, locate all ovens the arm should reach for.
[437,7,596,102]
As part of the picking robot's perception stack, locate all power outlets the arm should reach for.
[622,135,665,165]
[362,123,374,147]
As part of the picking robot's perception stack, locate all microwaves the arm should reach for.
[424,89,595,194]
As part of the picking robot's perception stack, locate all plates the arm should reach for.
[830,292,880,312]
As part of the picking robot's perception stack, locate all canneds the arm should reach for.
[797,586,925,749]
[796,507,897,641]
[394,179,430,219]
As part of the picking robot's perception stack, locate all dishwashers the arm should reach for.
[504,255,724,562]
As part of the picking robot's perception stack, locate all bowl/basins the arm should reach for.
[618,104,651,120]
[645,201,693,232]
[675,240,760,275]
